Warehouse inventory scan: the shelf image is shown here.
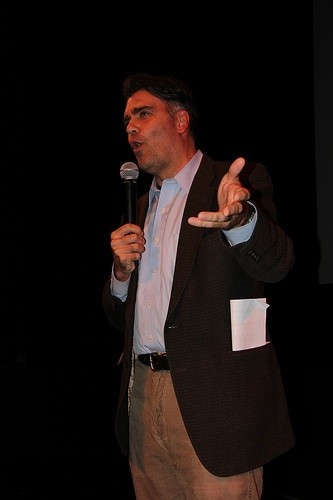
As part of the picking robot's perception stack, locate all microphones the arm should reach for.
[119,162,140,223]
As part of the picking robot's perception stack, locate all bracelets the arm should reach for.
[242,210,255,227]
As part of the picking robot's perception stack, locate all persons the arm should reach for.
[103,72,295,500]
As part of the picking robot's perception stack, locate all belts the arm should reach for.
[137,352,169,371]
